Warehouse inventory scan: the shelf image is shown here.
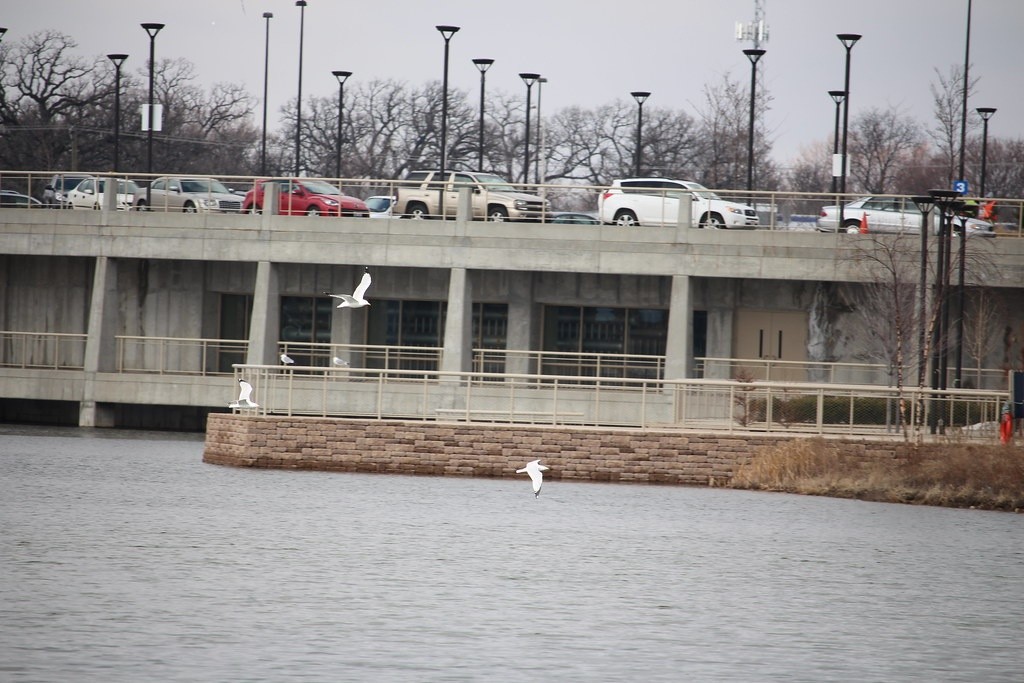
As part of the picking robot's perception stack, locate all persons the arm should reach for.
[963,194,978,217]
[983,192,998,223]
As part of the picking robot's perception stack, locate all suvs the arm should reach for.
[43,174,94,209]
[393,168,552,222]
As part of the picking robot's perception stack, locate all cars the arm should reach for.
[243,178,370,218]
[363,197,402,219]
[132,177,246,214]
[597,177,760,229]
[0,190,43,208]
[66,177,137,212]
[536,211,600,225]
[817,196,996,237]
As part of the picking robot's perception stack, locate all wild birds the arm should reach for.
[515,460,550,497]
[226,378,260,408]
[332,355,352,367]
[321,266,372,309]
[278,351,297,365]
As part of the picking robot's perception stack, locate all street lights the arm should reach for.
[828,90,850,205]
[976,106,997,196]
[108,22,354,211]
[913,190,980,435]
[631,92,651,178]
[742,49,766,207]
[436,25,461,220]
[471,58,494,172]
[837,33,862,233]
[519,72,541,193]
